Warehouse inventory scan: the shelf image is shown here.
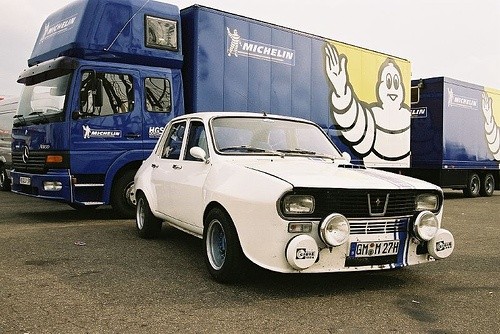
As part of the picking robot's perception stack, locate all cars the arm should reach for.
[130,112,456,286]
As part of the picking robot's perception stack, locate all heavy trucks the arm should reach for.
[407,77,500,195]
[10,0,413,222]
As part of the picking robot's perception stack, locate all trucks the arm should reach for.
[0,92,63,195]
